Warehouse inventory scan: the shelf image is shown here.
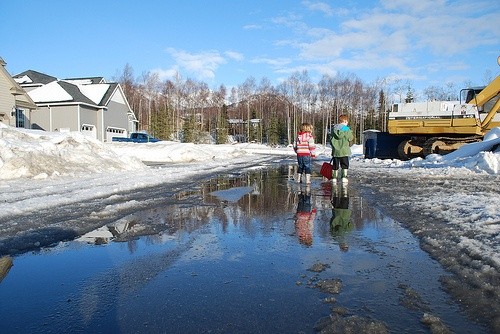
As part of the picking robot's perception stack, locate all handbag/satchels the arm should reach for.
[320,161,333,181]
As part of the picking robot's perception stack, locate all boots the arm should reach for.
[332,169,339,183]
[294,173,302,183]
[305,174,312,184]
[341,168,348,183]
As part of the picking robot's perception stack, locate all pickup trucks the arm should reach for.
[112,133,159,142]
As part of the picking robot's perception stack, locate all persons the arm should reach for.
[293,123,315,184]
[329,114,354,182]
[295,184,316,247]
[330,182,354,252]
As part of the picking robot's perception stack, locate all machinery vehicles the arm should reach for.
[386,55,500,160]
